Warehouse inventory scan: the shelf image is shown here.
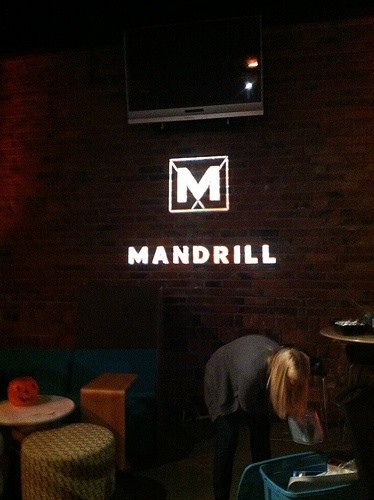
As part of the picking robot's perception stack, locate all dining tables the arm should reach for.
[320,326,374,344]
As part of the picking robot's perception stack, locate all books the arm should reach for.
[287,410,325,445]
[286,457,360,492]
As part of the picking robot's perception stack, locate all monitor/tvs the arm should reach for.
[124,0,264,123]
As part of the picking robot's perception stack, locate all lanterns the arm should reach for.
[8,377,38,407]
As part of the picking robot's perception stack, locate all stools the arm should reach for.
[20,422,117,500]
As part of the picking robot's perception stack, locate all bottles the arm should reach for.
[371,313,374,335]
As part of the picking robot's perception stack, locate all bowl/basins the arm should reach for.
[332,320,369,336]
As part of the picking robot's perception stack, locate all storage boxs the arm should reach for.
[236,451,352,500]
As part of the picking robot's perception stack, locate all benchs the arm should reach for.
[0,345,158,455]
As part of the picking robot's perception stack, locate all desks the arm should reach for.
[0,395,76,435]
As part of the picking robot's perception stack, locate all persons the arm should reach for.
[204,333,310,500]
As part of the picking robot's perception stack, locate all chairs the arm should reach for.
[341,344,374,443]
[280,345,332,444]
